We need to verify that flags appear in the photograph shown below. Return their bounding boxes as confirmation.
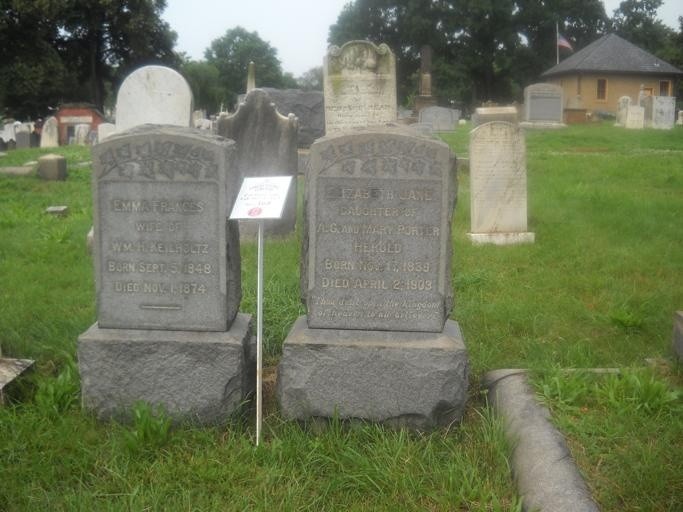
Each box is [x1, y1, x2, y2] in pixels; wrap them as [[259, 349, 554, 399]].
[[559, 34, 573, 52]]
